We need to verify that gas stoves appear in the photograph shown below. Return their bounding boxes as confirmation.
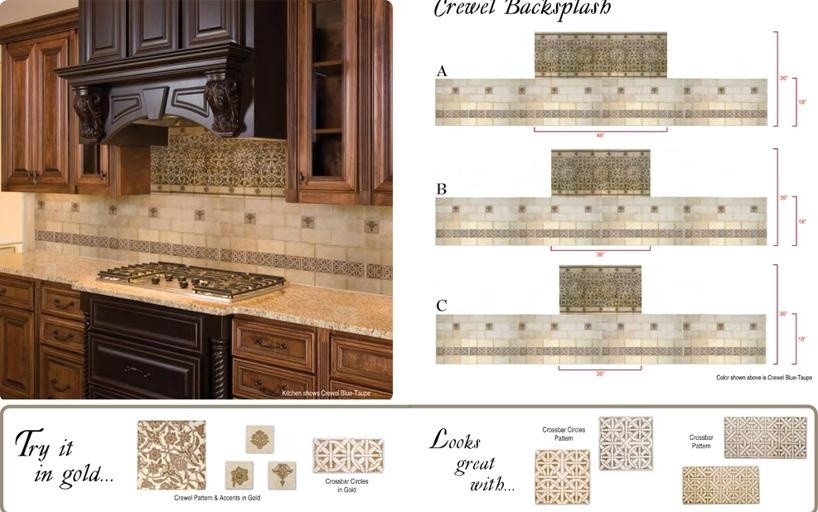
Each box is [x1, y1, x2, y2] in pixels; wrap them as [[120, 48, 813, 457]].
[[96, 260, 287, 304]]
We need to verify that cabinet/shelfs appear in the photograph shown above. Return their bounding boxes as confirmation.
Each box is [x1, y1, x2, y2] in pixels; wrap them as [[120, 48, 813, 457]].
[[0, 273, 392, 401], [0, 0, 393, 206]]
